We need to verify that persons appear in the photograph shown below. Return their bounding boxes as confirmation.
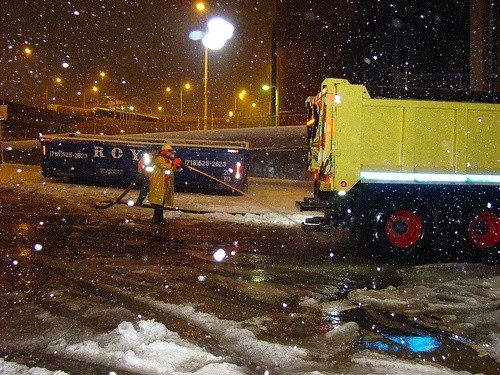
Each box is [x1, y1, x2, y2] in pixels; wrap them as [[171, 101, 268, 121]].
[[148, 143, 182, 227], [135, 148, 159, 208]]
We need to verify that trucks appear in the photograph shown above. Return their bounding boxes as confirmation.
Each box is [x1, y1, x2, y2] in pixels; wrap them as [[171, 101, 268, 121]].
[[295, 78, 500, 264]]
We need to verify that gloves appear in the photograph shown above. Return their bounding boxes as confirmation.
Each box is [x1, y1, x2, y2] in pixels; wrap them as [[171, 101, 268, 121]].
[[173, 158, 181, 167]]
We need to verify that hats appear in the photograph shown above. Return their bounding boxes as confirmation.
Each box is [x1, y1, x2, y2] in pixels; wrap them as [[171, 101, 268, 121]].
[[161, 144, 172, 150]]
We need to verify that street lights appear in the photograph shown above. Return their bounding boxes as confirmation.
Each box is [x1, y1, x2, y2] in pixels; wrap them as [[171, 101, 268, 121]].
[[196, 2, 210, 138]]
